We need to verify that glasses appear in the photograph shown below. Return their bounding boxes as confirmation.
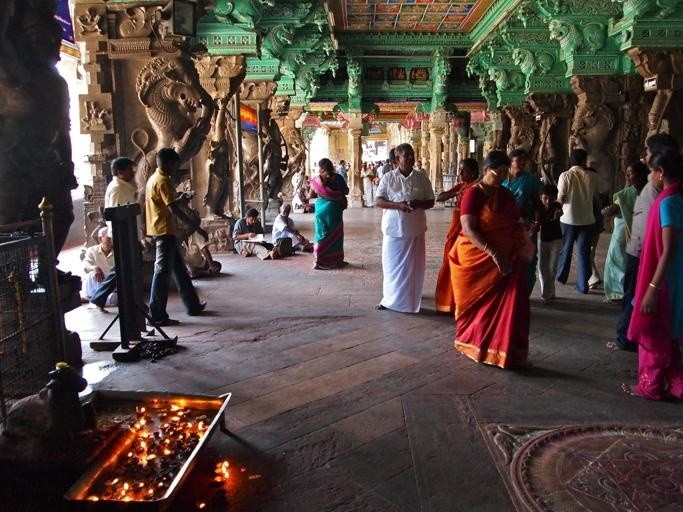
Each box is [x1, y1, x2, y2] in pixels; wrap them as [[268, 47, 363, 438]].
[[489, 168, 509, 179]]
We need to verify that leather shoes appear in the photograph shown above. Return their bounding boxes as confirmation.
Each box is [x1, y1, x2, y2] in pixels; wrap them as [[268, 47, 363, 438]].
[[147, 318, 180, 327], [187, 300, 207, 316]]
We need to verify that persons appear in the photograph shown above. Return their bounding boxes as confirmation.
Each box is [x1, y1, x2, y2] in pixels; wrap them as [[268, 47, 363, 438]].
[[529, 184, 564, 302], [374, 143, 435, 313], [413, 159, 428, 175], [308, 158, 349, 269], [291, 148, 399, 214], [555, 150, 610, 295], [435, 147, 543, 370], [271, 203, 314, 253], [82, 148, 222, 327], [601, 132, 683, 401], [232, 209, 279, 260]]
